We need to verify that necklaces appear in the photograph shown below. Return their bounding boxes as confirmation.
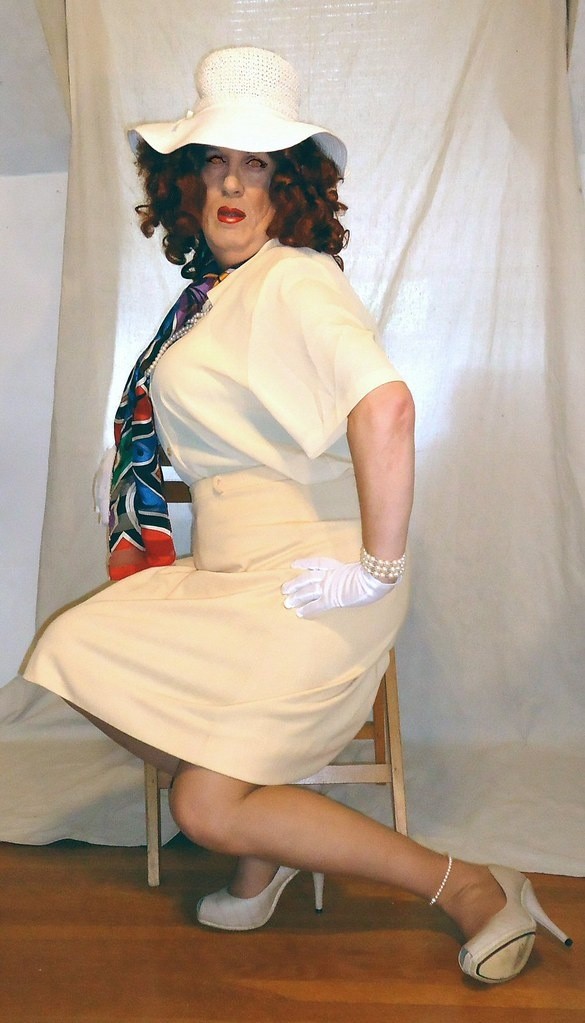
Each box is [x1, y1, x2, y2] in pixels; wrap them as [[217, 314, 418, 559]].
[[146, 304, 212, 377]]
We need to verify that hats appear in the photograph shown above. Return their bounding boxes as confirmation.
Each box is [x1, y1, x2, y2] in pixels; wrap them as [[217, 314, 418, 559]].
[[128, 46, 347, 183]]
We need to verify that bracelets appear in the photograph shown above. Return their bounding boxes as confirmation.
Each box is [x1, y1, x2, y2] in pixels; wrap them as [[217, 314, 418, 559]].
[[360, 546, 406, 578]]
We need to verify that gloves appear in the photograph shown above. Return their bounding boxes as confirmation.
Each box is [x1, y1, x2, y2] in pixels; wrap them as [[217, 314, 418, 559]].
[[280, 553, 402, 619]]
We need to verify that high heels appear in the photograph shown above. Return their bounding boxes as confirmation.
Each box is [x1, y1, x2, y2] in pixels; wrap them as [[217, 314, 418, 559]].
[[457, 868, 572, 983], [196, 865, 323, 930]]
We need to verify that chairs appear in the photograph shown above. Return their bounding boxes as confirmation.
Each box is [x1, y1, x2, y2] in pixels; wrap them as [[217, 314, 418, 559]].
[[147, 444, 406, 889]]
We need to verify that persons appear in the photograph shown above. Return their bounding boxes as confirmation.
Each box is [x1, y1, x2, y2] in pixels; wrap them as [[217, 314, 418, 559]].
[[22, 48, 571, 982]]
